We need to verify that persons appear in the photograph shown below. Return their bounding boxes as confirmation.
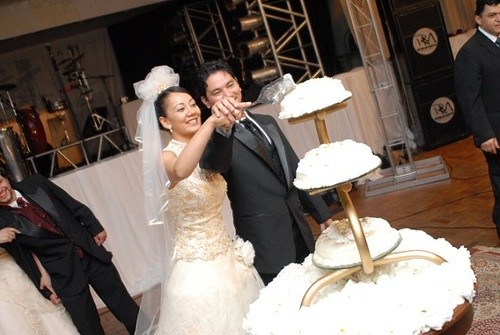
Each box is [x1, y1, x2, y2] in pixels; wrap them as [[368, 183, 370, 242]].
[[134, 65, 266, 335], [453, 0, 500, 241], [0, 227, 81, 334], [199, 58, 335, 287], [0, 167, 156, 335]]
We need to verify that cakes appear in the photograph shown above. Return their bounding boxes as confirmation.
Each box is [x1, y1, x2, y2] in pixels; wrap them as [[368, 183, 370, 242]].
[[313, 217, 400, 267], [278, 76, 352, 120], [293, 139, 382, 190]]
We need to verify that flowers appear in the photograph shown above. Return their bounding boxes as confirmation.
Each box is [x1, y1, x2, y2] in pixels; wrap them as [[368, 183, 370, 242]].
[[235, 238, 256, 265]]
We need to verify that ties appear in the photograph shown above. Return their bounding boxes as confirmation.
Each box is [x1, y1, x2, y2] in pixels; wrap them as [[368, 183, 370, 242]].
[[496, 38, 500, 45], [241, 117, 285, 175], [16, 198, 28, 206]]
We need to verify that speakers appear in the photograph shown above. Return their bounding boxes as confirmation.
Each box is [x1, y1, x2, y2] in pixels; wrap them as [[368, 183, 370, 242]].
[[376, 0, 473, 151]]
